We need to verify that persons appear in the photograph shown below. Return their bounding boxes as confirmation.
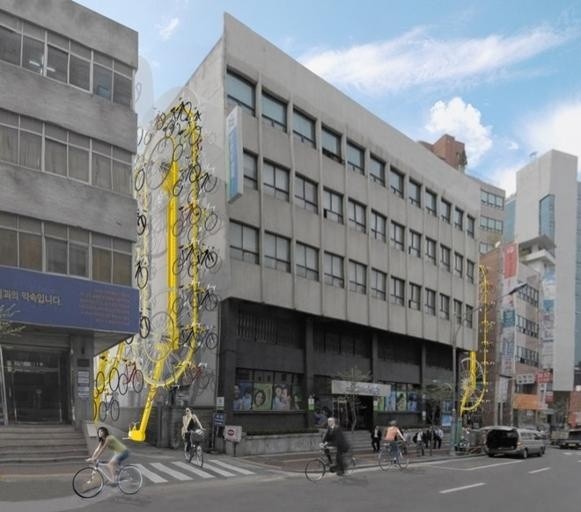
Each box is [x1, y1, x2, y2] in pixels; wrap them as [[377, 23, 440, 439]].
[[314, 410, 328, 441], [181, 407, 206, 454], [234, 384, 291, 410], [319, 417, 348, 475], [370, 420, 444, 467], [87, 428, 128, 485]]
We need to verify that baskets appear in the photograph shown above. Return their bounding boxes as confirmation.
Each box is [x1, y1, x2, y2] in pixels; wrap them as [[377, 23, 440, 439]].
[[190, 434, 206, 443]]
